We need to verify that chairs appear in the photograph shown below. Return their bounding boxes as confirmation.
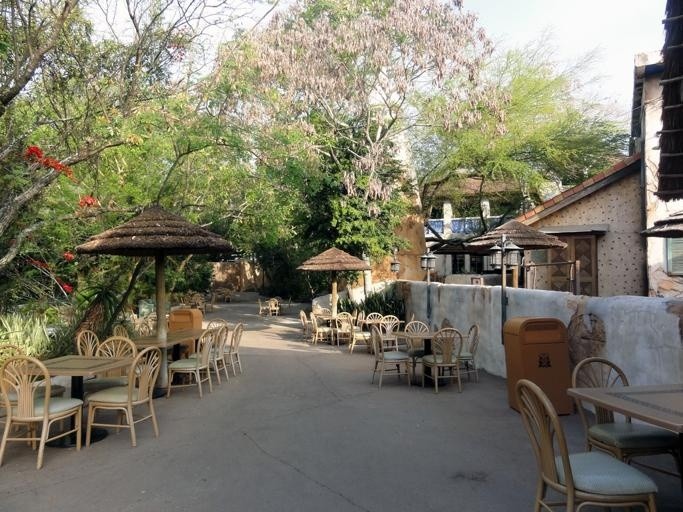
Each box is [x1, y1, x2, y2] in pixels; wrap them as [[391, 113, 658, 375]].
[[514, 378, 659, 512], [77, 318, 245, 448], [571, 357, 682, 491], [300, 308, 480, 394], [0, 344, 66, 447], [178, 285, 233, 315], [0, 354, 84, 470], [256, 295, 282, 318]]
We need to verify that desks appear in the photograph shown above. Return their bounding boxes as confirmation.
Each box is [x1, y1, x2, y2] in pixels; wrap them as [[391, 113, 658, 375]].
[[6, 354, 145, 447], [566, 384, 682, 434]]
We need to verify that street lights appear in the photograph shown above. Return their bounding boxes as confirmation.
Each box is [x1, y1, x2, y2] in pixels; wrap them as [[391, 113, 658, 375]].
[[389, 255, 401, 282], [417, 248, 437, 325], [488, 232, 523, 346]]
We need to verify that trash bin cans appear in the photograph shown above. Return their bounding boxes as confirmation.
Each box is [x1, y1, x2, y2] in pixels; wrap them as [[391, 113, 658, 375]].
[[503, 316, 575, 415], [168, 309, 202, 345]]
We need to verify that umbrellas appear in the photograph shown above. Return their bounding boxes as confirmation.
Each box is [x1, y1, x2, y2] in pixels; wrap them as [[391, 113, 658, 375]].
[[468, 218, 568, 289], [296, 247, 372, 340], [74, 204, 235, 388]]
